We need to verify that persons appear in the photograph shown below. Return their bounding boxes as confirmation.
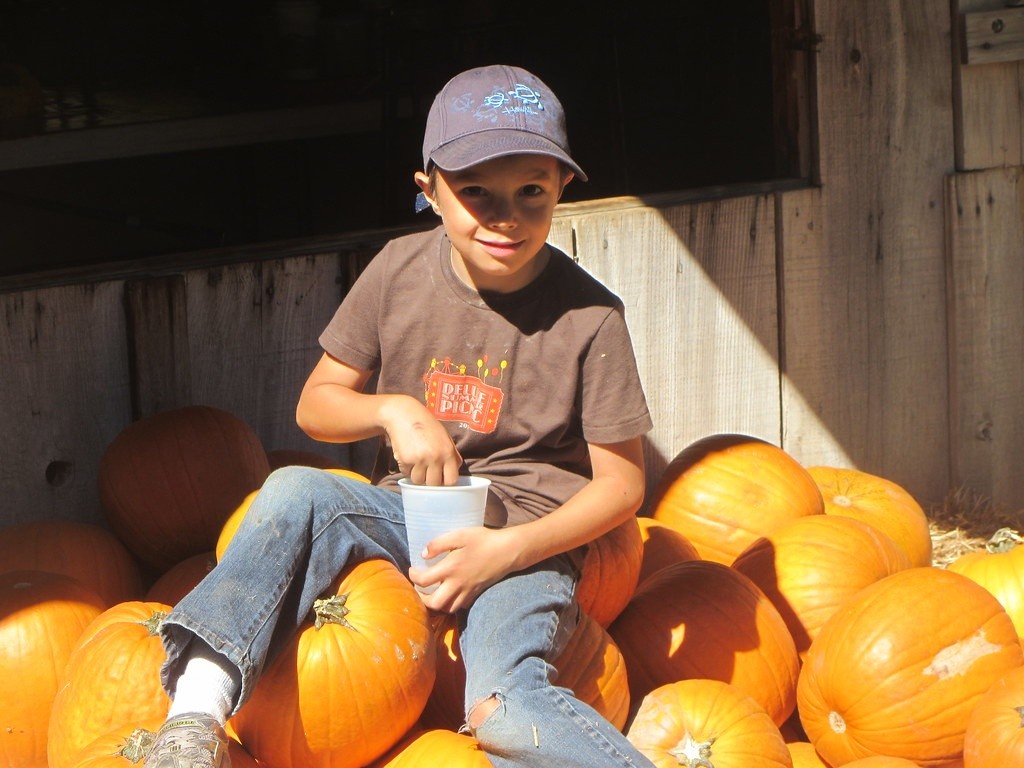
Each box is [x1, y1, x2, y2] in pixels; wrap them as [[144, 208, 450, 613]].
[[120, 65, 660, 768]]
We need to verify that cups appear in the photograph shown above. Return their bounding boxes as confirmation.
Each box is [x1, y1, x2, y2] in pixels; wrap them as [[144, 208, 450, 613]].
[[399, 476, 491, 593]]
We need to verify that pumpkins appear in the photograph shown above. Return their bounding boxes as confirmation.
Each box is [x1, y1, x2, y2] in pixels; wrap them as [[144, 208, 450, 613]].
[[0, 406, 1023, 767]]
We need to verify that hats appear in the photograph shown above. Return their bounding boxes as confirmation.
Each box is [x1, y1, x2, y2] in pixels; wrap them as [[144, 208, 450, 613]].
[[422, 64, 588, 182]]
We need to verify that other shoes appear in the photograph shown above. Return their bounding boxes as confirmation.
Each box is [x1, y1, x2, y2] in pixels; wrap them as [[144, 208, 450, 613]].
[[118, 712, 230, 768]]
